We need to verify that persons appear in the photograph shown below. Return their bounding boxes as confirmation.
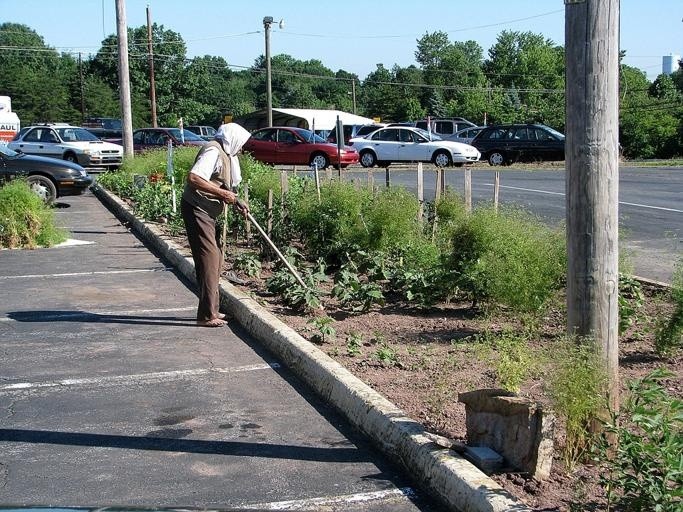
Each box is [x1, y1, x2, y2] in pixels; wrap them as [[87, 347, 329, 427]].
[[180, 123, 252, 327]]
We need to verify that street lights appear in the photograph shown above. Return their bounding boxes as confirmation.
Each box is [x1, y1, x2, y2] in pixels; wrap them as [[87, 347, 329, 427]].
[[262, 15, 286, 127]]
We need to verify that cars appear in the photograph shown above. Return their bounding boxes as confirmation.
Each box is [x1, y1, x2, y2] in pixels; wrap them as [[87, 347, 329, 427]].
[[0, 117, 218, 208]]
